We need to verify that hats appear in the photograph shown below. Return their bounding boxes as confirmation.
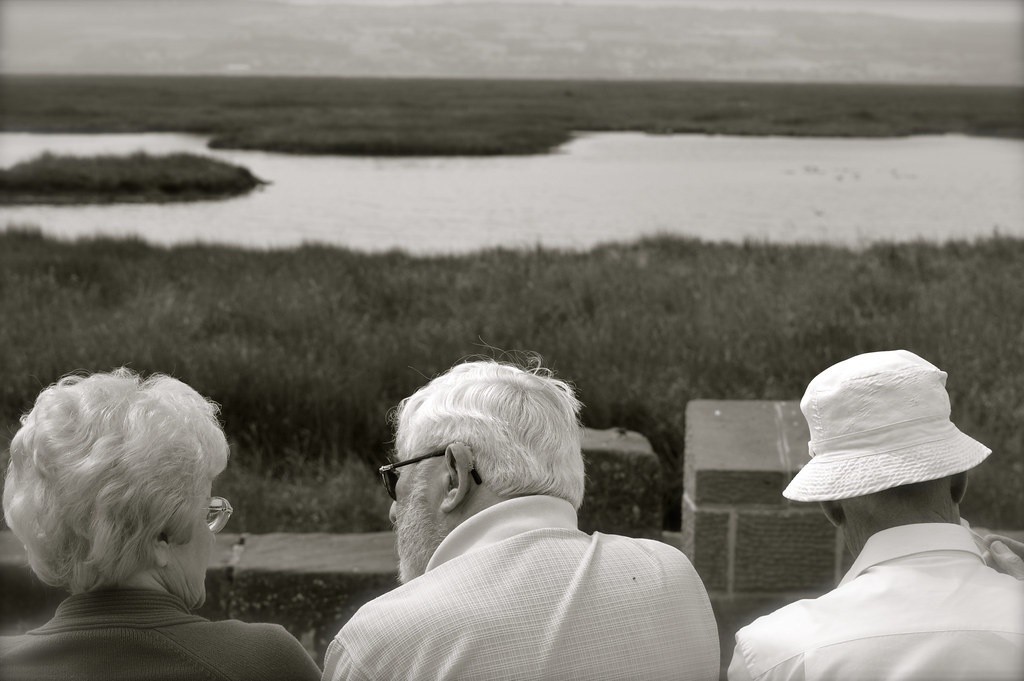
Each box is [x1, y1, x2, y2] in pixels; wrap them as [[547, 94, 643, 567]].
[[783, 349, 994, 501]]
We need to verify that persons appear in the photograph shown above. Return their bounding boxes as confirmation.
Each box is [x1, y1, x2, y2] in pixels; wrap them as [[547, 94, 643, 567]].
[[0, 366, 324, 681], [724, 345, 1022, 680], [311, 356, 721, 681]]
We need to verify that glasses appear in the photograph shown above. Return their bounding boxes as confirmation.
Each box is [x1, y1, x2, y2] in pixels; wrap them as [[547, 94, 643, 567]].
[[380, 448, 483, 501], [200, 496, 234, 533]]
